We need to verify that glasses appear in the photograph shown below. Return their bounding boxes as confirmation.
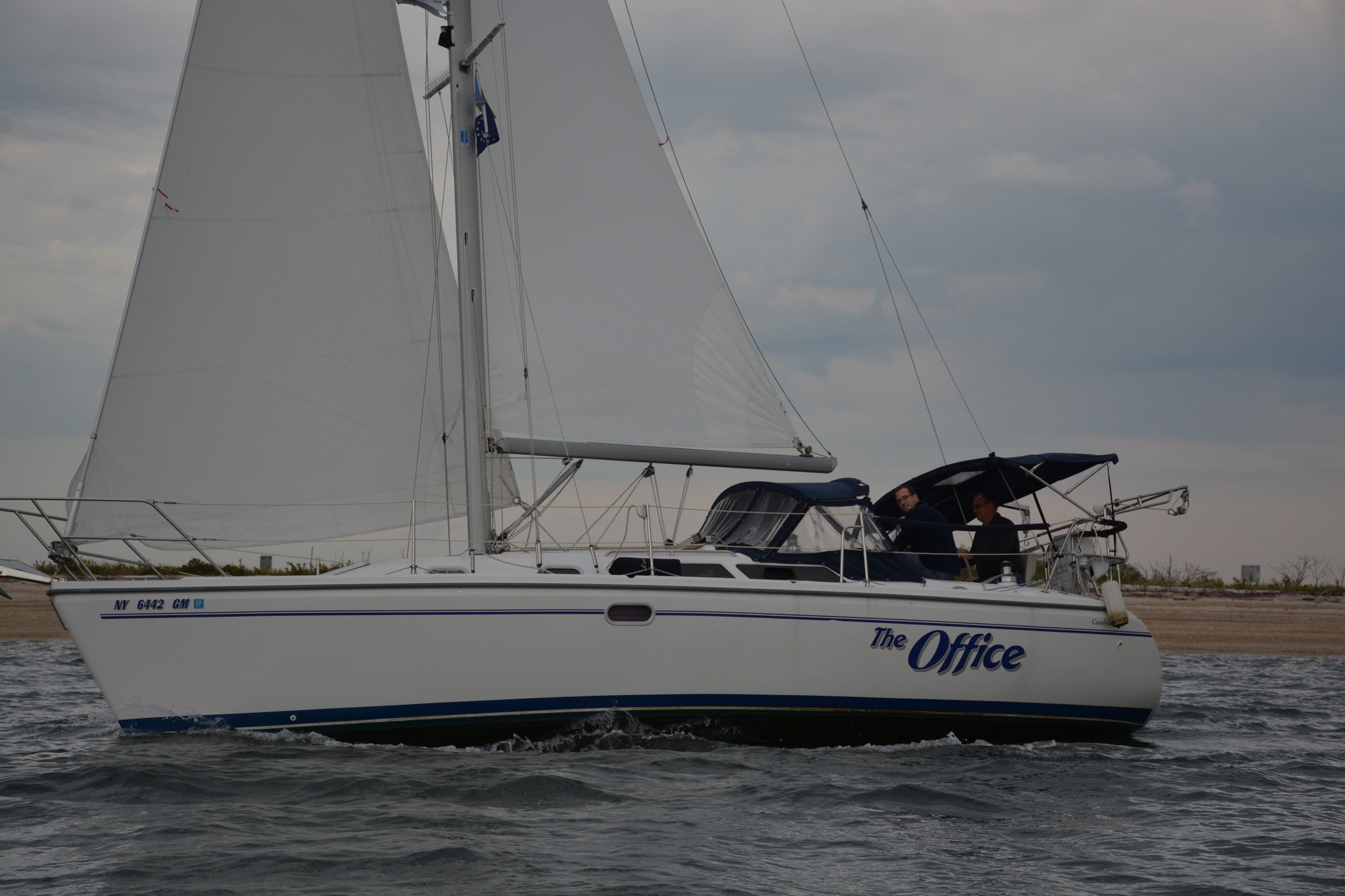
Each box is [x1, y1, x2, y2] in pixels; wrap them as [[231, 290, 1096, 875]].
[[896, 494, 913, 505], [973, 502, 992, 510]]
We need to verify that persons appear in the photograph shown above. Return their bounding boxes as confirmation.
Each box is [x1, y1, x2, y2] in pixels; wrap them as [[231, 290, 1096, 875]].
[[893, 485, 961, 580], [957, 492, 1024, 584]]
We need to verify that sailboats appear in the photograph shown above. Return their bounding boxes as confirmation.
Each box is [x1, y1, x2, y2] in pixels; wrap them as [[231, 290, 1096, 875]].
[[45, 1, 1193, 746]]
